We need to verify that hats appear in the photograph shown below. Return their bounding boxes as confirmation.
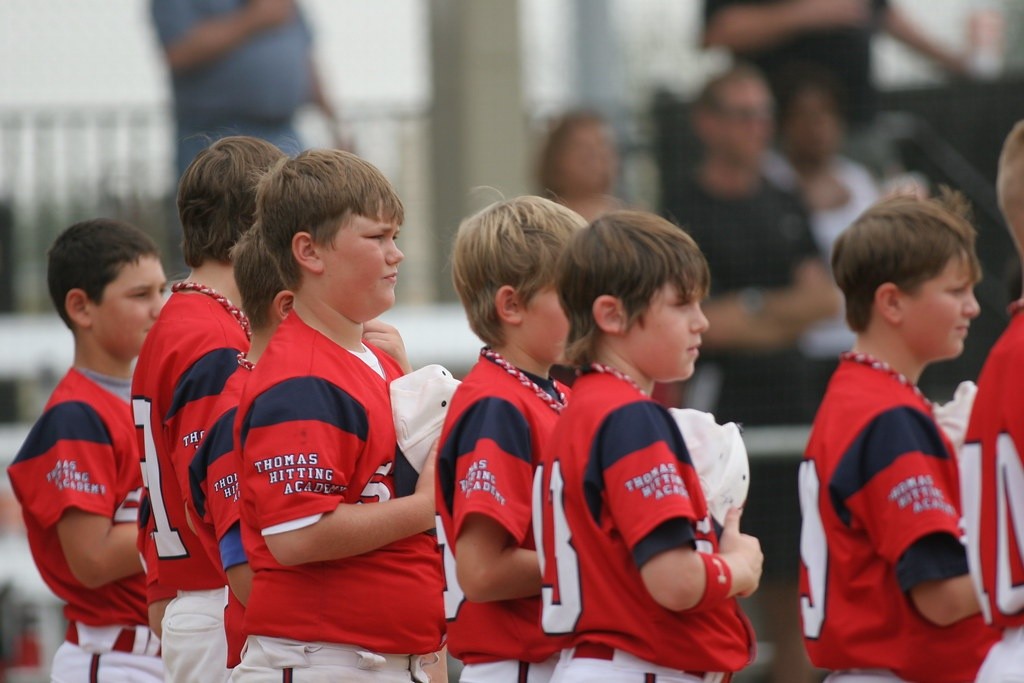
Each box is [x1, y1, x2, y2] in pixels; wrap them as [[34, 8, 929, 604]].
[[390, 363, 465, 536], [669, 405, 752, 543]]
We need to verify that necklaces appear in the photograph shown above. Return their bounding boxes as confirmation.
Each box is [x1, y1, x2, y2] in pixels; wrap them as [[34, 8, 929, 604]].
[[236, 352, 257, 370], [576, 362, 647, 397], [844, 352, 933, 405], [170, 282, 252, 341], [481, 346, 568, 410]]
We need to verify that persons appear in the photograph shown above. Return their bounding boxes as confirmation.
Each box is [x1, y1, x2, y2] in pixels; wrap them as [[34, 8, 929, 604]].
[[0, 0, 1024, 683], [801, 196, 981, 683]]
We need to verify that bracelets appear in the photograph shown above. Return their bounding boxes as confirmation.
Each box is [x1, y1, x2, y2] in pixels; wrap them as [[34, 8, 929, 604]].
[[683, 551, 730, 617]]
